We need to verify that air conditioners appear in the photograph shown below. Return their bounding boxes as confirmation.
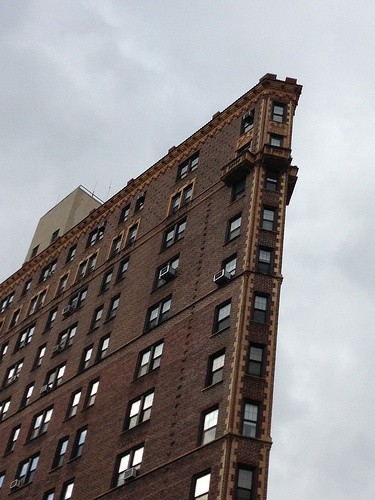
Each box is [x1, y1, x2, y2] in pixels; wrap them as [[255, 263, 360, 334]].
[[7, 375, 19, 385], [39, 384, 50, 395], [214, 269, 230, 283], [123, 468, 137, 480], [45, 272, 52, 279], [242, 112, 253, 120], [136, 192, 145, 200], [52, 342, 62, 354], [63, 305, 71, 315], [9, 478, 21, 491], [159, 265, 176, 281]]
